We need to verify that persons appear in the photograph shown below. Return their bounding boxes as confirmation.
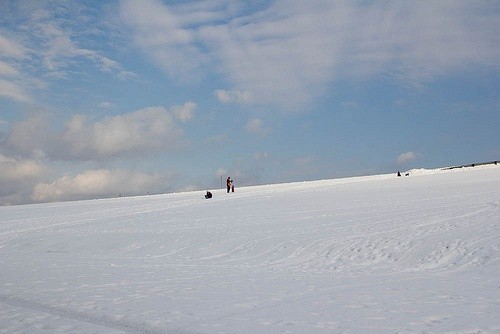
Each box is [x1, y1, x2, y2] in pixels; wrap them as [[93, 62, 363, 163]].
[[397, 160, 499, 177], [226, 176, 231, 193], [205, 191, 212, 199], [231, 179, 234, 192]]
